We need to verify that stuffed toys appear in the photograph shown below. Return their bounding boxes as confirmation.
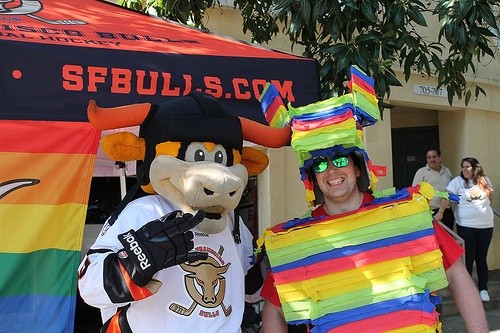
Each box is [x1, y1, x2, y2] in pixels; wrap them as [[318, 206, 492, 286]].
[[78, 99, 292, 333]]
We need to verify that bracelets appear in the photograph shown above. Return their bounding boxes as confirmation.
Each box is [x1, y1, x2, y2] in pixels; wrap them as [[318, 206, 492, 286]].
[[438, 209, 446, 215]]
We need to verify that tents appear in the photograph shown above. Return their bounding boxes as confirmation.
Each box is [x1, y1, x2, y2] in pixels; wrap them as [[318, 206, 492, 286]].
[[0, 0, 320, 333]]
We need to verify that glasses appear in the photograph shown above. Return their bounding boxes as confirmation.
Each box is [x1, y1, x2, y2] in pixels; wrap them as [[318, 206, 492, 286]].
[[314, 157, 348, 173]]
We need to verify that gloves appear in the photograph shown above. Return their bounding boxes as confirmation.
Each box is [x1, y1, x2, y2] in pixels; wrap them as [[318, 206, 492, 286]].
[[116, 209, 209, 287]]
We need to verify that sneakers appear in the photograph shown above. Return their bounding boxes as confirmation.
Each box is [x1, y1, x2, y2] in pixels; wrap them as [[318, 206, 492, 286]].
[[479, 290, 490, 301]]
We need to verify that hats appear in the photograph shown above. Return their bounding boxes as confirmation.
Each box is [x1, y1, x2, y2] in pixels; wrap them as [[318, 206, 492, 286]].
[[291, 93, 379, 201]]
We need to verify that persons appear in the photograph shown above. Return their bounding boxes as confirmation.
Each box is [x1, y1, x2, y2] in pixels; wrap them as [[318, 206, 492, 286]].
[[258, 64, 494, 333]]
